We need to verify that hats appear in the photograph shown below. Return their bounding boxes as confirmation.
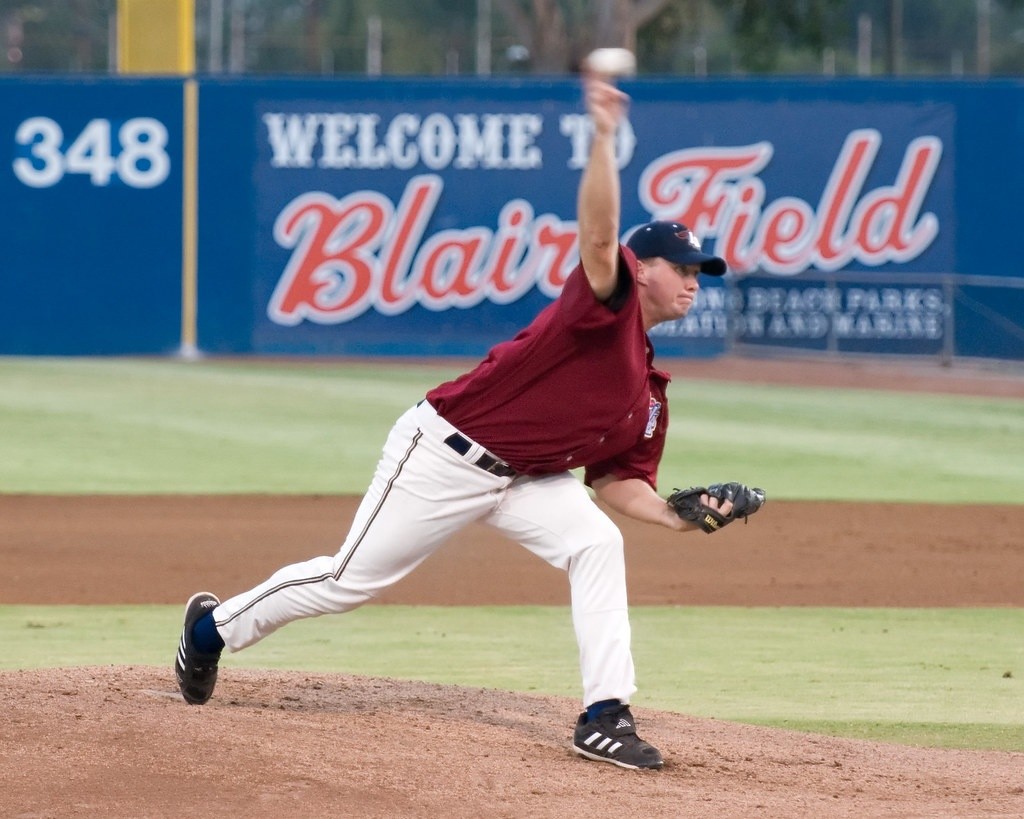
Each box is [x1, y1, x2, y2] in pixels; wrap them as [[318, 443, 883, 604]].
[[628, 220, 729, 277]]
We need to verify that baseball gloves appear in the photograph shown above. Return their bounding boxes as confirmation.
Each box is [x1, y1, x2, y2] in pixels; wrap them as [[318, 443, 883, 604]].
[[666, 482, 764, 534]]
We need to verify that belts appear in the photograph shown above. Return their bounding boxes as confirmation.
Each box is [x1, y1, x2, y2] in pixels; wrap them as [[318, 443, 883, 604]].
[[415, 399, 518, 478]]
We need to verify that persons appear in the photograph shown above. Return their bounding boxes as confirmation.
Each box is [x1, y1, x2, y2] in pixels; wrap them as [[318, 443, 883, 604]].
[[175, 59, 735, 769]]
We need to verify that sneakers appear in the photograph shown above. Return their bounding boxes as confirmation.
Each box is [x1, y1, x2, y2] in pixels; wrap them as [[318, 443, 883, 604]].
[[174, 592, 224, 705], [573, 702, 664, 773]]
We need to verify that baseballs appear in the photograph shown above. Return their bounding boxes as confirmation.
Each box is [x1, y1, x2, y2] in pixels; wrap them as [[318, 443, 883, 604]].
[[579, 43, 636, 79]]
